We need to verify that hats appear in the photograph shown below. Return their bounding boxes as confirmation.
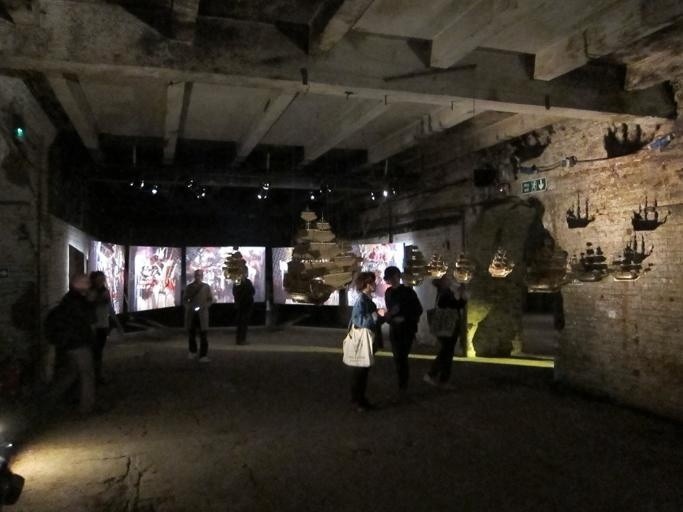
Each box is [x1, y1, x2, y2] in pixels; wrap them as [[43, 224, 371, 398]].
[[70, 274, 92, 290]]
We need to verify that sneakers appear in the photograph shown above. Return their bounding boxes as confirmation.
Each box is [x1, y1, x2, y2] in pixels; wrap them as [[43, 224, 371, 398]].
[[189, 352, 196, 359], [198, 355, 209, 362], [440, 384, 455, 390], [423, 374, 437, 386]]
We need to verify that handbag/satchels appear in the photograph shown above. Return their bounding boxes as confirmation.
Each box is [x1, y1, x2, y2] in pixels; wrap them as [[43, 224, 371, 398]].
[[342, 327, 376, 369], [426, 306, 458, 337]]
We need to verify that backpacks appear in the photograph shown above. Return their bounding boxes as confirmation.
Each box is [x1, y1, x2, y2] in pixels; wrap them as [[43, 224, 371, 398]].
[[44, 292, 80, 342]]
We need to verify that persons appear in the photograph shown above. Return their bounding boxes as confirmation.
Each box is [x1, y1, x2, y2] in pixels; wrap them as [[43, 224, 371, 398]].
[[423, 274, 469, 389], [384, 267, 423, 404], [91, 269, 125, 383], [343, 271, 400, 413], [232, 279, 256, 345], [182, 270, 212, 363], [39, 273, 104, 418]]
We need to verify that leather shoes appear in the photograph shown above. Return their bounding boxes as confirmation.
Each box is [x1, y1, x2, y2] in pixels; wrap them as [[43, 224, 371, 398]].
[[349, 401, 364, 413]]
